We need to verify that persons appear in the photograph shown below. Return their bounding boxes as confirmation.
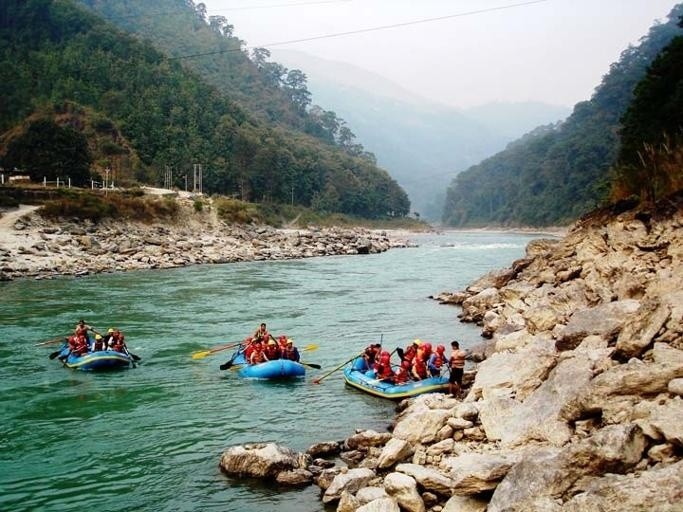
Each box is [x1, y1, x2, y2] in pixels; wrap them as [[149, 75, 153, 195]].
[[61, 318, 124, 353], [242, 323, 303, 364], [362, 341, 465, 401]]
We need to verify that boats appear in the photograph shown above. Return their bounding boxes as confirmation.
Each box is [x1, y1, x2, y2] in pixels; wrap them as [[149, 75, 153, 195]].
[[232, 346, 306, 380], [342, 355, 460, 399], [57, 334, 131, 371]]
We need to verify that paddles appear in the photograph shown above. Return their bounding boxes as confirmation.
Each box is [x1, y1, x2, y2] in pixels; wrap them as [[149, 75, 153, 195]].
[[120, 351, 140, 361], [299, 363, 322, 369], [57, 351, 74, 361], [298, 343, 319, 353], [123, 346, 137, 369], [220, 363, 249, 369], [397, 347, 407, 368], [49, 346, 68, 360], [193, 341, 245, 358], [35, 338, 65, 346]]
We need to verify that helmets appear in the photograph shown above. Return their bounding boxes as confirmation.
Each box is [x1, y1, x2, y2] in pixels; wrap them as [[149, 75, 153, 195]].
[[69, 336, 85, 343], [95, 329, 124, 340], [416, 359, 424, 366], [247, 337, 262, 350], [413, 338, 444, 354], [402, 360, 410, 369], [381, 351, 389, 363], [280, 336, 292, 346]]
